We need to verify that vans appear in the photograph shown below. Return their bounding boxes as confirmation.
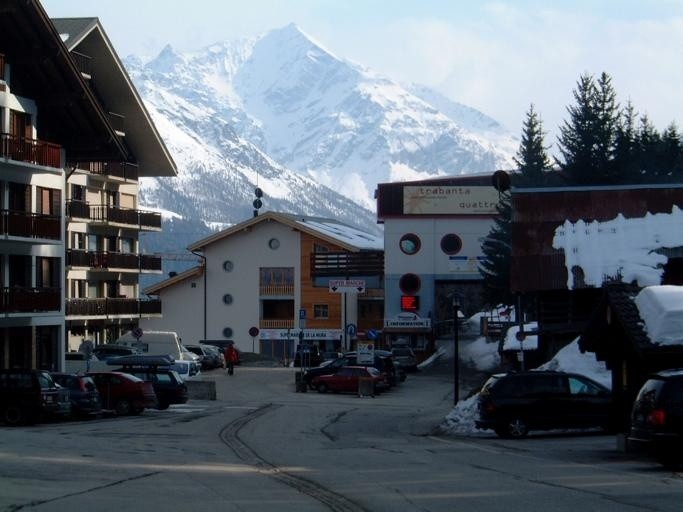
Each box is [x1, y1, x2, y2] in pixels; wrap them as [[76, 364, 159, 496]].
[[626, 368, 683, 467]]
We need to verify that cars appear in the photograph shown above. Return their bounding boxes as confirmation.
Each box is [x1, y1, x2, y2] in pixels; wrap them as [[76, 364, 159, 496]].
[[0, 329, 226, 426], [294, 345, 418, 394], [470, 371, 616, 439]]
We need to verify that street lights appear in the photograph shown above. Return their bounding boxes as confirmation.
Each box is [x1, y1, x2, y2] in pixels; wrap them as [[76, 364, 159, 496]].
[[446, 289, 467, 407]]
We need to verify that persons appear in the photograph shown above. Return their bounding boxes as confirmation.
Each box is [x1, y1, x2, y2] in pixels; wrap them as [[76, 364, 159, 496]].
[[223, 339, 238, 376]]
